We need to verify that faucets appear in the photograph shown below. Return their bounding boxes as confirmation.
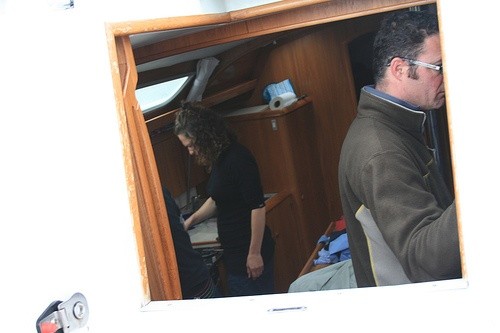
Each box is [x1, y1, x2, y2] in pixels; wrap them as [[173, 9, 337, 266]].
[[192, 194, 201, 210]]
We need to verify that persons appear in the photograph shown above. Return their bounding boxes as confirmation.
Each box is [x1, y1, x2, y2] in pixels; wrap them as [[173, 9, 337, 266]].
[[337, 7, 462, 289], [174, 102, 275, 300], [158, 181, 219, 299]]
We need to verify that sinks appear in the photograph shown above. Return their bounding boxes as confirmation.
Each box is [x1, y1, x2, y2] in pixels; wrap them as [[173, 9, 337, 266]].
[[207, 193, 273, 222]]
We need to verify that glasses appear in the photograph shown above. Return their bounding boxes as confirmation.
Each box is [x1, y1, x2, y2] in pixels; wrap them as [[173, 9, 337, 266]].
[[386, 56, 442, 77]]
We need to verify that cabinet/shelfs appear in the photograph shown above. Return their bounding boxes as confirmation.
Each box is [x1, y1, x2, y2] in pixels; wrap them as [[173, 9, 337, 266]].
[[187, 97, 354, 299]]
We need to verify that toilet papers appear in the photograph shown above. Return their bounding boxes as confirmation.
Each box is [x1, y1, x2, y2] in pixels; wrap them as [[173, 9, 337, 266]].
[[269, 92, 297, 111]]
